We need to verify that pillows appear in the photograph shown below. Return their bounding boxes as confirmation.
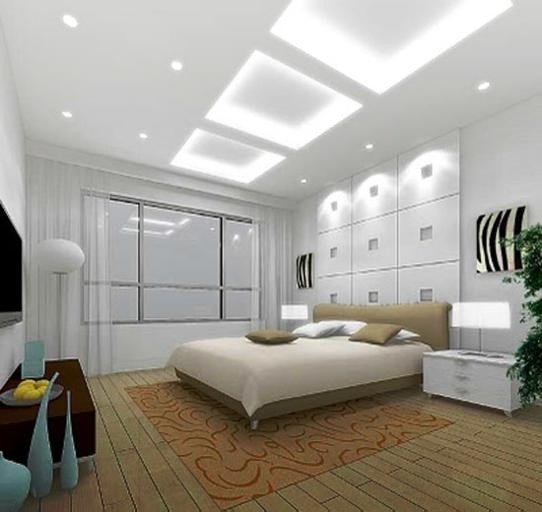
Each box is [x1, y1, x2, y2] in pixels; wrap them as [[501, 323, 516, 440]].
[[292, 320, 421, 346], [245, 329, 300, 345]]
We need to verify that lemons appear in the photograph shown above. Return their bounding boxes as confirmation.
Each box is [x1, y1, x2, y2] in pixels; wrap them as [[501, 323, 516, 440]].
[[14, 378, 49, 401]]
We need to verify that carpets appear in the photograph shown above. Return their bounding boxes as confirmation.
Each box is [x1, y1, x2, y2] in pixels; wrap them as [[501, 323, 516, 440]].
[[124, 379, 457, 512]]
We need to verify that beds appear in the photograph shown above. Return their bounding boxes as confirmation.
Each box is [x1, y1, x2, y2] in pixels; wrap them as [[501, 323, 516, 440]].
[[165, 302, 453, 432]]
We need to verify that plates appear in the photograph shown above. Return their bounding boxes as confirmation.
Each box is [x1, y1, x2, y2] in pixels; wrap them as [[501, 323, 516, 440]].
[[1, 385, 64, 406]]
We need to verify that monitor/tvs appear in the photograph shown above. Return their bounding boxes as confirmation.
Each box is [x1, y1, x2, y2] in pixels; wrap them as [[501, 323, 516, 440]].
[[0, 201, 23, 328]]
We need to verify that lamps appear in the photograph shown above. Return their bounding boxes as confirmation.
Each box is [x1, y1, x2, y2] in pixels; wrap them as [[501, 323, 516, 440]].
[[451, 301, 511, 358], [34, 238, 85, 360], [281, 304, 308, 333]]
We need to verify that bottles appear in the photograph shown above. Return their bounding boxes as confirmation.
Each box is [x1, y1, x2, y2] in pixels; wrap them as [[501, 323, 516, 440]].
[[60, 391, 81, 489], [0, 451, 31, 512], [24, 371, 60, 498]]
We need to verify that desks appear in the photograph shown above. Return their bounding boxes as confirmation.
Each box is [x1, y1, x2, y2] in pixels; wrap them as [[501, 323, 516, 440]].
[[0, 357, 98, 478]]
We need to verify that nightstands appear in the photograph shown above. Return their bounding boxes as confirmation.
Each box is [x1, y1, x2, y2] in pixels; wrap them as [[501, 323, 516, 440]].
[[421, 348, 537, 419]]
